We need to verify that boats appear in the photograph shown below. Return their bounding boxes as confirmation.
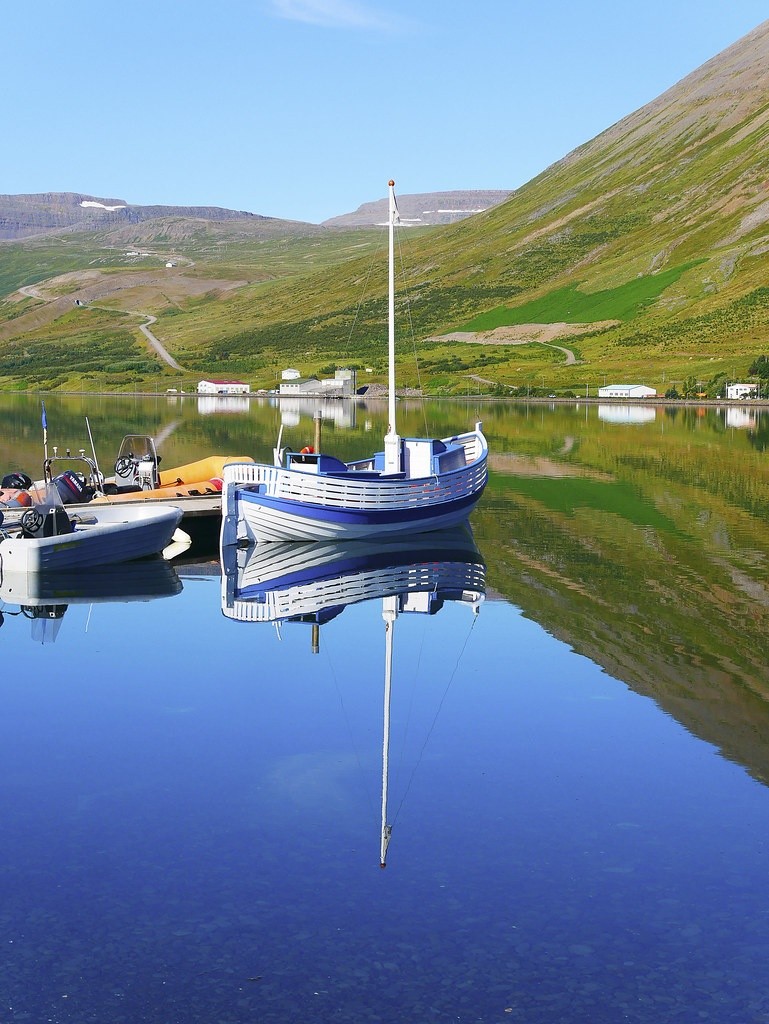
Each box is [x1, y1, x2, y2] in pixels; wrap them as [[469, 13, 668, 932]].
[[11, 556, 184, 604], [10, 505, 181, 568], [0, 453, 254, 504]]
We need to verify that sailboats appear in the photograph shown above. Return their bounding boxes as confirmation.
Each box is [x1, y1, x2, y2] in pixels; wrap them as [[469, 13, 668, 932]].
[[200, 521, 488, 867], [220, 181, 491, 544]]
